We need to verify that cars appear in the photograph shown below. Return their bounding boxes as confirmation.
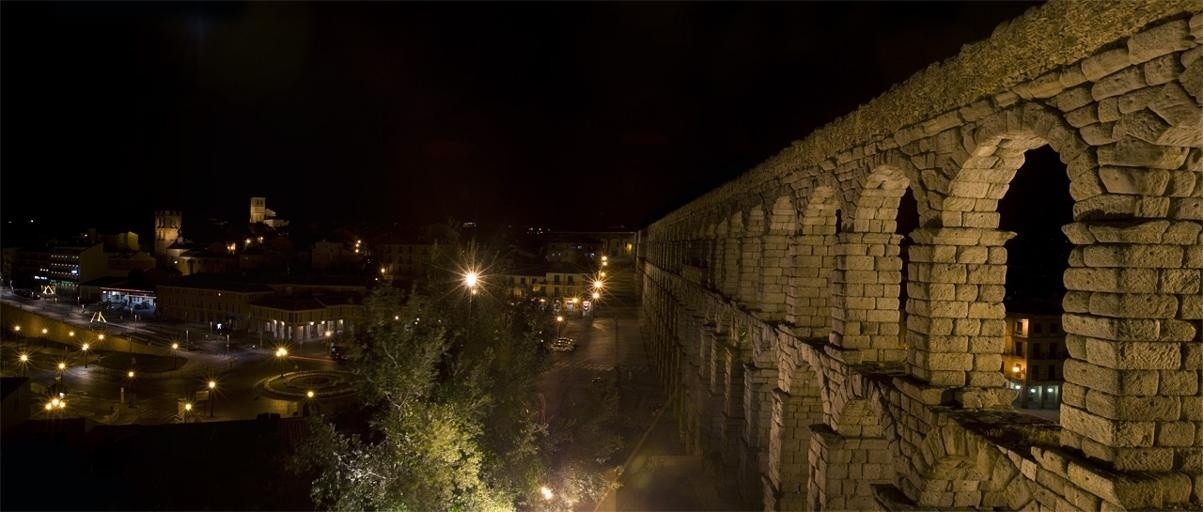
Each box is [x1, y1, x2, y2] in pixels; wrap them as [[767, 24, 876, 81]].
[[550, 336, 576, 353]]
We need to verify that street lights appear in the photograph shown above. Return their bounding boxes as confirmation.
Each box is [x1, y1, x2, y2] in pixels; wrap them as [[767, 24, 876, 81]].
[[208, 381, 216, 417], [58, 362, 66, 392], [557, 316, 563, 336], [274, 346, 288, 380], [14, 325, 106, 354], [19, 354, 28, 375], [128, 371, 136, 409], [81, 342, 89, 369], [45, 398, 65, 418], [171, 342, 178, 370], [593, 292, 599, 318]]
